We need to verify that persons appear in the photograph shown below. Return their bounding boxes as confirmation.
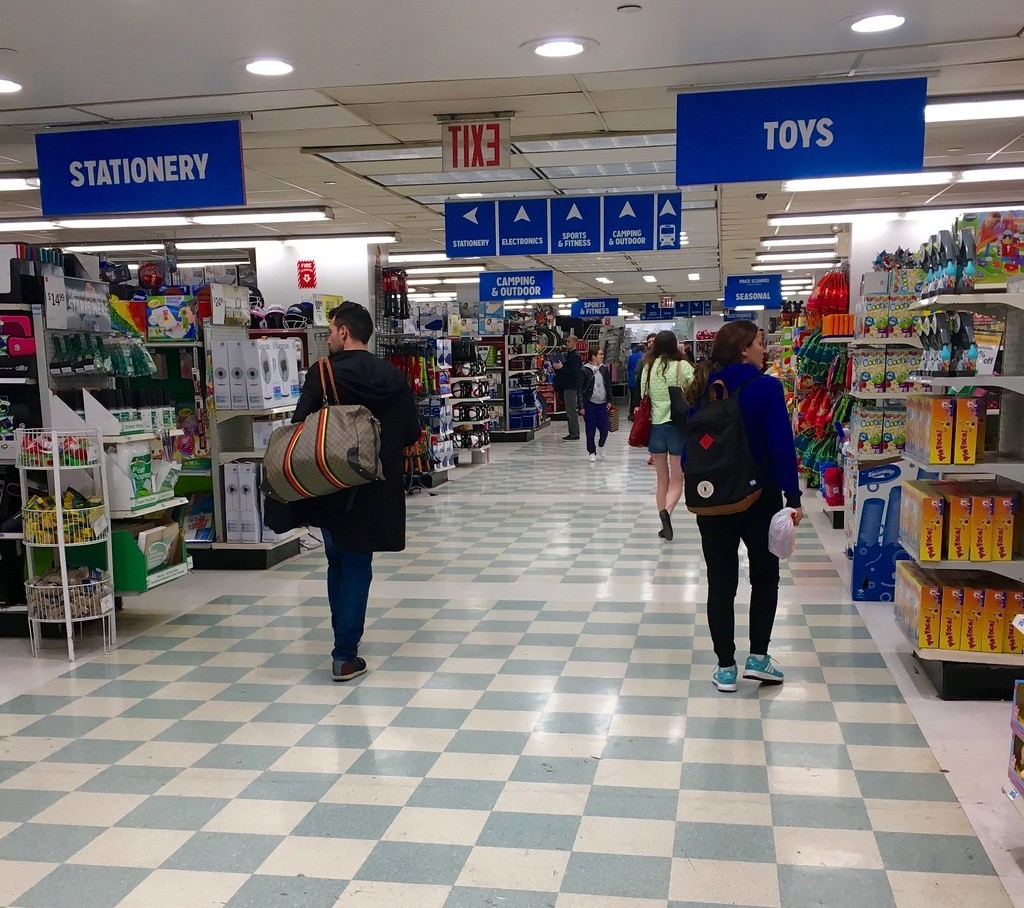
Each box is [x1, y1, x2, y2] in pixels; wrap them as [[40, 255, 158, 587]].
[[681, 320, 803, 692], [640, 331, 695, 541], [685, 345, 695, 363], [759, 329, 768, 372], [292, 301, 421, 681], [553, 335, 582, 442], [627, 344, 647, 421], [577, 347, 613, 462], [636, 333, 656, 464]]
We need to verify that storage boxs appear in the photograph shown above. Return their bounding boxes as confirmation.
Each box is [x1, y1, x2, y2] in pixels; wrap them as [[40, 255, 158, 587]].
[[251, 418, 292, 454], [906, 396, 986, 465], [224, 460, 294, 542], [895, 477, 1024, 653], [211, 338, 300, 411]]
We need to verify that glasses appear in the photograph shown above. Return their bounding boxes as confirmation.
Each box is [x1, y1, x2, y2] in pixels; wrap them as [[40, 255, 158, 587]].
[[647, 340, 654, 344], [597, 352, 604, 355]]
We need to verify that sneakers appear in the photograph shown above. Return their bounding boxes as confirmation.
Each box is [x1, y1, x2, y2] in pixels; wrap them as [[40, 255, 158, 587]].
[[742, 652, 784, 682], [712, 664, 738, 692]]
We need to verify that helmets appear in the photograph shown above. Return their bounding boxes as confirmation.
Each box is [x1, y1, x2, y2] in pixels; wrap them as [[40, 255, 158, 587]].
[[99, 257, 313, 328]]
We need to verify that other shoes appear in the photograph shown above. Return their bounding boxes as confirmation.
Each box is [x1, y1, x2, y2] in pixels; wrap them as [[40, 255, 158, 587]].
[[646, 457, 655, 465], [660, 509, 673, 541], [597, 438, 605, 458], [562, 435, 580, 441], [589, 453, 597, 462], [332, 657, 367, 681], [659, 530, 666, 539]]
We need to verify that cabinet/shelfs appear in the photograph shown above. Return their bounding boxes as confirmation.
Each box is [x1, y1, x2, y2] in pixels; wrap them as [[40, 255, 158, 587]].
[[0, 302, 194, 634], [905, 277, 1024, 666], [444, 333, 551, 451], [843, 293, 925, 558], [119, 320, 306, 568]]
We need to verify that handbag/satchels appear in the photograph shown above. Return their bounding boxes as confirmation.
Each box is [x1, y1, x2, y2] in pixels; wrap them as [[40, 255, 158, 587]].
[[576, 391, 587, 417], [608, 406, 619, 433], [264, 355, 386, 503], [768, 507, 799, 560], [628, 365, 651, 448], [668, 359, 688, 424]]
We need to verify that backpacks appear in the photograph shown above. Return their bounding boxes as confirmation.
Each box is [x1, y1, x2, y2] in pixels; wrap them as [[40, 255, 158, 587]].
[[680, 373, 777, 515]]
